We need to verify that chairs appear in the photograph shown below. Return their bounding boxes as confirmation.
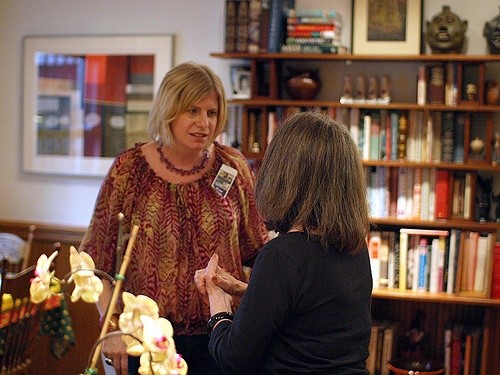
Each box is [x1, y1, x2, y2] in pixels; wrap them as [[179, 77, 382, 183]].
[[0, 241, 62, 375]]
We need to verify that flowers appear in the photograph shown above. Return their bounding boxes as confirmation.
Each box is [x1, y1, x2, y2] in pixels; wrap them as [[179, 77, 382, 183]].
[[397, 311, 434, 375], [29, 245, 188, 375]]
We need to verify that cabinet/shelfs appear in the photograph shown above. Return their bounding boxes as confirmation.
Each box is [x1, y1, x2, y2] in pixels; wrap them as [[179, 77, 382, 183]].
[[210, 53, 500, 306]]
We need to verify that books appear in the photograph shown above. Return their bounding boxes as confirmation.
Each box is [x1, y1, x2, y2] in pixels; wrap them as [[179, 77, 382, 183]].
[[225, 0, 500, 375]]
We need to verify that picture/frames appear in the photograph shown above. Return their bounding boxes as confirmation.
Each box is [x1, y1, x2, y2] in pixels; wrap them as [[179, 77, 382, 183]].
[[18, 32, 177, 180], [350, 0, 424, 55]]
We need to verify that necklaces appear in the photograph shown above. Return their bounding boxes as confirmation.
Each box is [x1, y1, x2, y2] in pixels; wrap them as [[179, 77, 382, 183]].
[[156, 139, 209, 175]]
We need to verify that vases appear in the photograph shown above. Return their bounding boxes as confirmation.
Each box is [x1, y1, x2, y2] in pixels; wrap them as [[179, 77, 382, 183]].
[[386, 361, 446, 375]]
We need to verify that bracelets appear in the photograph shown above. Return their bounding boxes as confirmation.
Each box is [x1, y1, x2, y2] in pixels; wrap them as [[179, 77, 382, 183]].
[[99, 313, 119, 333], [207, 311, 234, 336]]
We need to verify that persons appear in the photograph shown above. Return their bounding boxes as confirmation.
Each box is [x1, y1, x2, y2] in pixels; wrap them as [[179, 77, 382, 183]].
[[194, 113, 372, 375], [81, 63, 270, 375]]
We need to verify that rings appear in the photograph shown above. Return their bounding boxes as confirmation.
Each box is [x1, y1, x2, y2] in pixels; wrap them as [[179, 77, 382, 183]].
[[104, 358, 113, 366]]
